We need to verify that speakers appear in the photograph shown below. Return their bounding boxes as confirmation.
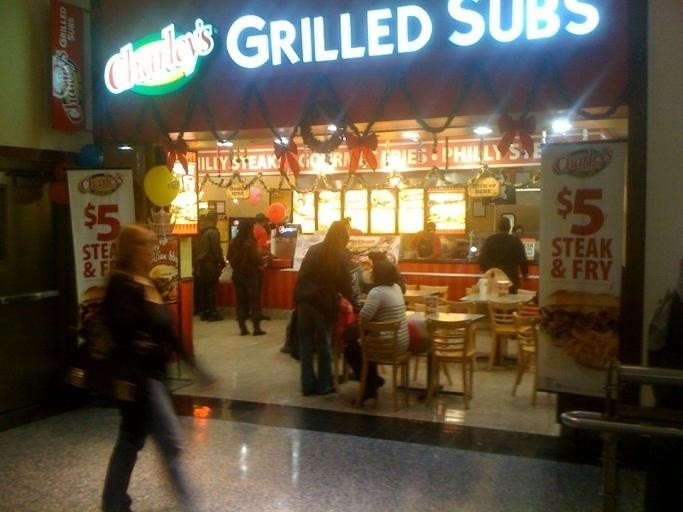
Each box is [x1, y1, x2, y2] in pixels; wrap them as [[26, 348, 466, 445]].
[[482, 184, 515, 205]]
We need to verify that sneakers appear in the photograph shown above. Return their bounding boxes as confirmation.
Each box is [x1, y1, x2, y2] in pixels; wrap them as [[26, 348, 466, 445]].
[[206, 311, 224, 323]]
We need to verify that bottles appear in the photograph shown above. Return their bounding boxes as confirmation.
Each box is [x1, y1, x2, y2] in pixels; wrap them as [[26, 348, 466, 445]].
[[471, 285, 477, 296]]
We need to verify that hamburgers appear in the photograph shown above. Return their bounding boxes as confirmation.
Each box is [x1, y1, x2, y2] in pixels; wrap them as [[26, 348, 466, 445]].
[[538, 290, 621, 347], [80, 286, 107, 312]]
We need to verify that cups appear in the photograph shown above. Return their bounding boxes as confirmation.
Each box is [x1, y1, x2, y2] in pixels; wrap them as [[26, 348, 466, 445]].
[[478, 278, 489, 299], [413, 303, 426, 319], [495, 279, 510, 297]]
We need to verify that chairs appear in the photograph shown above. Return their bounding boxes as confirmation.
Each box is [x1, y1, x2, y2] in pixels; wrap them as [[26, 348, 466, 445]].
[[322, 282, 549, 415]]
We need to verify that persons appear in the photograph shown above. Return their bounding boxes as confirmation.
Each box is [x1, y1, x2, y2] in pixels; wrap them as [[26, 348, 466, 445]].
[[194, 209, 225, 322], [279, 215, 410, 407], [94, 222, 218, 512], [225, 218, 266, 336], [409, 220, 442, 260], [191, 214, 206, 316], [510, 224, 523, 237], [251, 213, 271, 251], [478, 216, 529, 340]]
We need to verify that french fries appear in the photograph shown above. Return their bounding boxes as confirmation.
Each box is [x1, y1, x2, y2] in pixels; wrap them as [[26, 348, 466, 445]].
[[565, 330, 620, 368]]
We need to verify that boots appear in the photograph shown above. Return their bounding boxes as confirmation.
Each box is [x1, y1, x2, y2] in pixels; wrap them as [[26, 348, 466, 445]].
[[252, 321, 267, 336], [237, 321, 250, 335]]
